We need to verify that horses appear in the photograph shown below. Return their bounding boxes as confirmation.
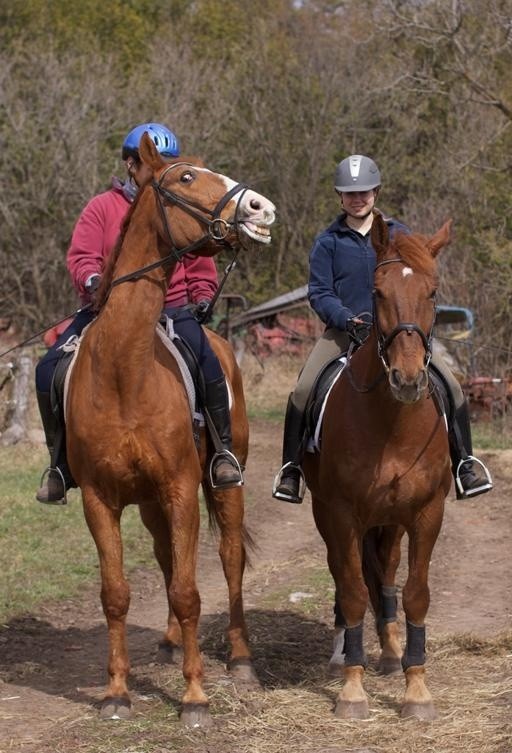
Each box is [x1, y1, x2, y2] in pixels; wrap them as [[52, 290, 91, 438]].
[[58, 130, 264, 730], [295, 208, 454, 722]]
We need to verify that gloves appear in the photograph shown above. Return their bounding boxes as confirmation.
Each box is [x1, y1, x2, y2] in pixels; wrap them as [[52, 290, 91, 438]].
[[195, 300, 215, 324], [349, 319, 369, 338]]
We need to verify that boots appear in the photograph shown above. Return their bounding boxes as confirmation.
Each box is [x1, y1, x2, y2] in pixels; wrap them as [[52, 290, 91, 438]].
[[447, 388, 491, 501], [273, 388, 308, 502], [201, 370, 242, 485], [32, 390, 80, 501]]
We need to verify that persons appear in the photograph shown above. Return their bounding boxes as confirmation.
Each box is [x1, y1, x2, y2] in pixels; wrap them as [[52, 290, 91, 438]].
[[272, 154, 493, 504], [35, 124, 243, 503]]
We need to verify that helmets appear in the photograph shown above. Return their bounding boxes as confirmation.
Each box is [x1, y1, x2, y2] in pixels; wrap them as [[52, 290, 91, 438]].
[[118, 121, 182, 161], [333, 154, 382, 195]]
